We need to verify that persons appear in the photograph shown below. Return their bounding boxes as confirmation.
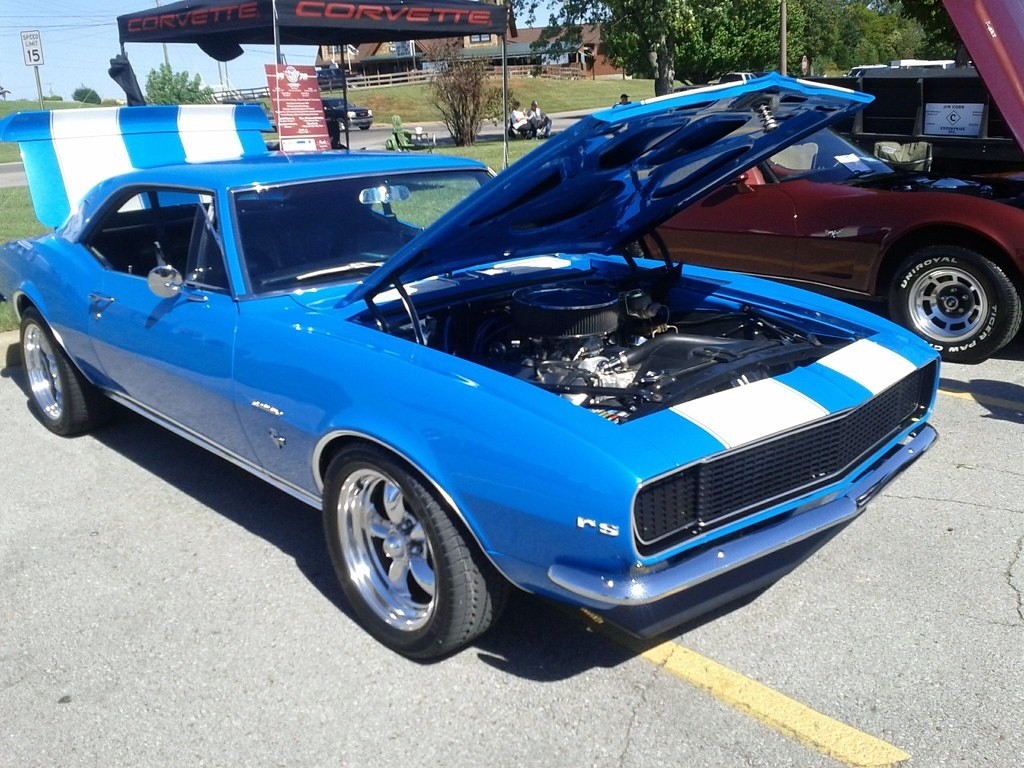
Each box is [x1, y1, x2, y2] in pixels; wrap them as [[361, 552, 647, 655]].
[[612, 94, 631, 108], [510, 100, 552, 140]]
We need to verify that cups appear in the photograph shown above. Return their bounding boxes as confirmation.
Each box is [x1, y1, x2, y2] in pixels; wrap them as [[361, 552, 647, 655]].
[[415, 127, 422, 133]]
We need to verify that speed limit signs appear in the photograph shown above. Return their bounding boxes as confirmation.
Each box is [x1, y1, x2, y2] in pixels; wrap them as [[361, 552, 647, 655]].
[[20, 30, 44, 66]]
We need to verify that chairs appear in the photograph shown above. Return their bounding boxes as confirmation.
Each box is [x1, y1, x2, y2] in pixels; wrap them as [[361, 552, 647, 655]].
[[391, 114, 437, 154]]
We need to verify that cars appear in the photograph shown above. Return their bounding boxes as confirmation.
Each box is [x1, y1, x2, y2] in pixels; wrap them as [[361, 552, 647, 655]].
[[0, 70, 940, 665], [842, 64, 887, 76], [319, 97, 374, 131], [626, 0, 1024, 366], [221, 99, 277, 132], [315, 71, 347, 92], [719, 72, 758, 81]]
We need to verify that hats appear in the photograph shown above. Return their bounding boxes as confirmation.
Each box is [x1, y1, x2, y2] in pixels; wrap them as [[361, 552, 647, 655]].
[[531, 100, 538, 106], [621, 93, 630, 98]]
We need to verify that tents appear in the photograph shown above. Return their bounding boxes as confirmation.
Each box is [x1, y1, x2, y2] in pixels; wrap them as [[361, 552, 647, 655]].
[[116, 0, 508, 169]]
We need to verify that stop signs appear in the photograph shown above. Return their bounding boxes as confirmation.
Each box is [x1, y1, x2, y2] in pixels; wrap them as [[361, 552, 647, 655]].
[[802, 55, 808, 75]]
